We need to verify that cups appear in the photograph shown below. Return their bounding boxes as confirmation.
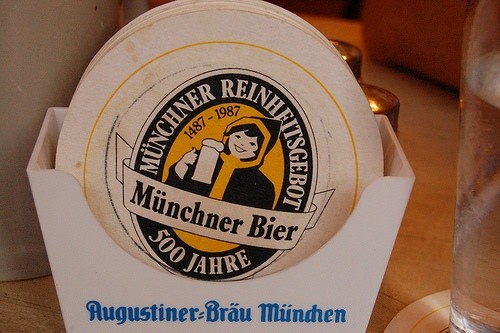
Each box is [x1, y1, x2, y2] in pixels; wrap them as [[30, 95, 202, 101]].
[[448, 0, 500, 333]]
